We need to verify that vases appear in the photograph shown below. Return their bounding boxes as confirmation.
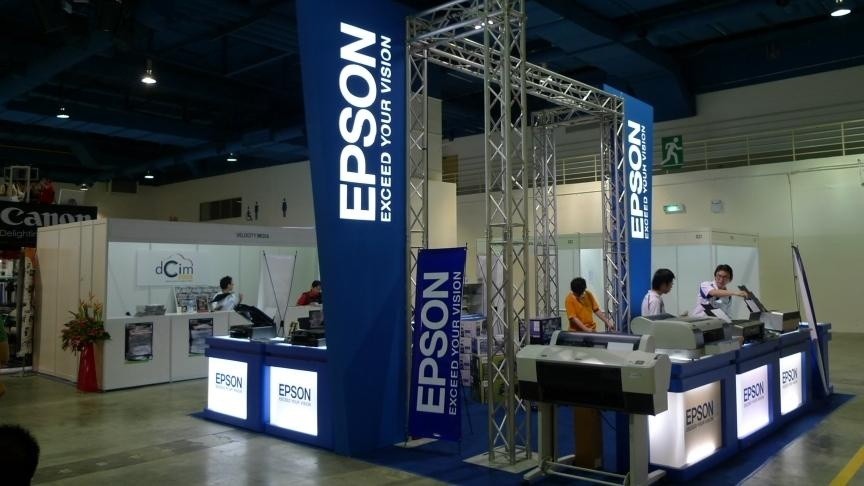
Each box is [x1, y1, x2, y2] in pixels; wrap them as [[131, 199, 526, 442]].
[[76, 344, 99, 393]]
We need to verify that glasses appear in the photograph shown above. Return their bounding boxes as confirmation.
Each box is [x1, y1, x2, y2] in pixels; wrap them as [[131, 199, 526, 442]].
[[716, 274, 728, 280]]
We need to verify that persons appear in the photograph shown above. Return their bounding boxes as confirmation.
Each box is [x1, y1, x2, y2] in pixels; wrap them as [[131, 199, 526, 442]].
[[296, 280, 322, 308], [659, 137, 683, 165], [694, 264, 748, 318], [641, 268, 676, 316], [211, 276, 244, 311], [565, 277, 614, 333], [0, 174, 55, 203], [0, 424, 44, 486]]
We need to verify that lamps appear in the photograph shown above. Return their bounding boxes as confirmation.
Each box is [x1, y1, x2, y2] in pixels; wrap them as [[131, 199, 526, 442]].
[[226, 152, 238, 163], [143, 168, 155, 179], [830, 0, 852, 18], [56, 103, 70, 118], [140, 58, 157, 85]]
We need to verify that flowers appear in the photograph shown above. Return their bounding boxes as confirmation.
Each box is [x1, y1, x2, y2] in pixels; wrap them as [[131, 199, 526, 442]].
[[60, 292, 112, 355]]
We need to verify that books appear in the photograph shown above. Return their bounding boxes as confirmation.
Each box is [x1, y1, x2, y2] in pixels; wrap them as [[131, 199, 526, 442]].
[[134, 300, 166, 316]]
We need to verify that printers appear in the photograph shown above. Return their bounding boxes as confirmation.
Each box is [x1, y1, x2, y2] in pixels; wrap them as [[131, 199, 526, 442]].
[[291, 309, 327, 347], [631, 313, 740, 355], [701, 303, 764, 345], [516, 330, 671, 416], [739, 284, 800, 335], [229, 303, 276, 340]]
